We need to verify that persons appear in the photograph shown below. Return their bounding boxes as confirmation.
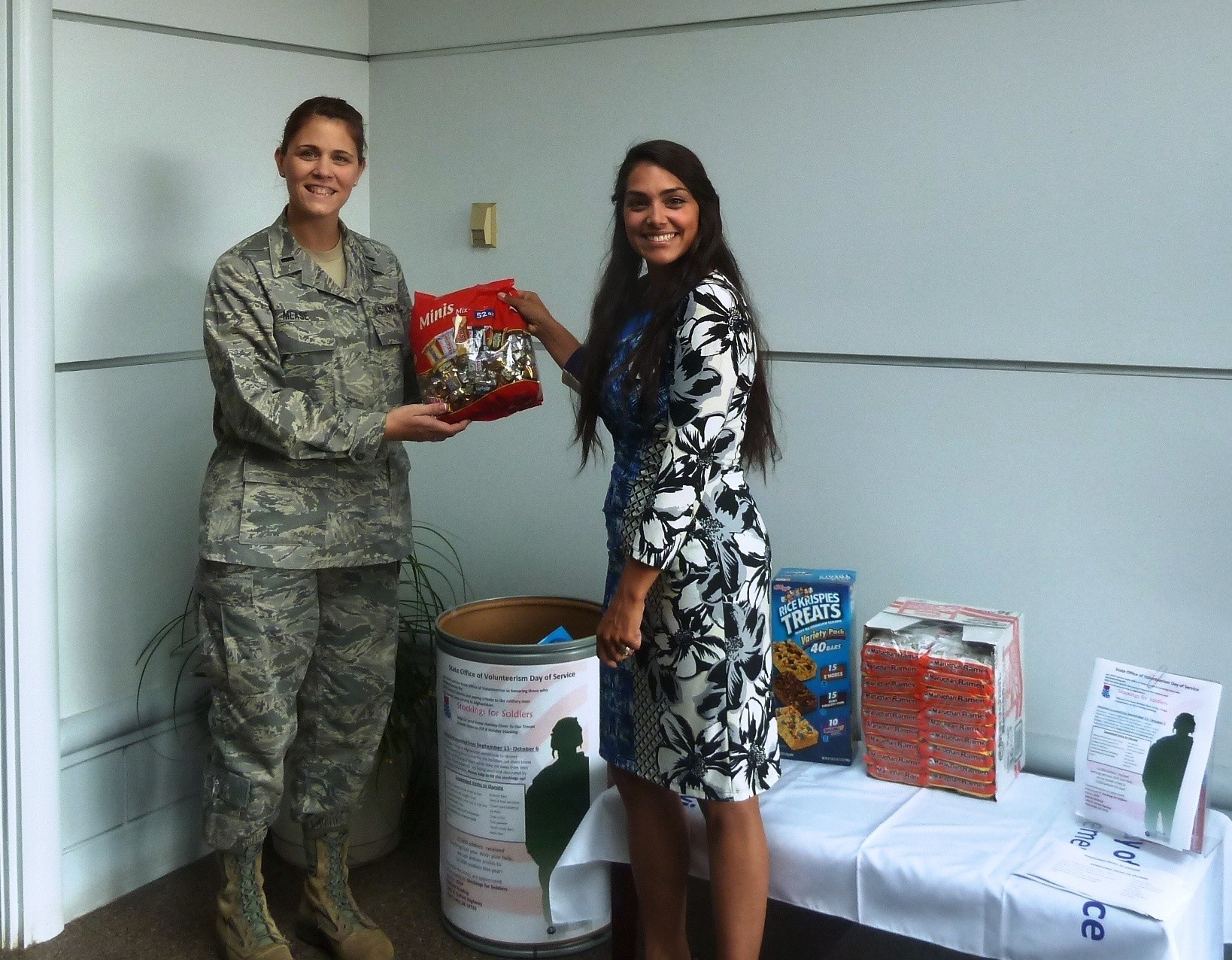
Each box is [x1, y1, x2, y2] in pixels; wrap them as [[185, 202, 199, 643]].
[[501, 141, 783, 960], [197, 95, 471, 960]]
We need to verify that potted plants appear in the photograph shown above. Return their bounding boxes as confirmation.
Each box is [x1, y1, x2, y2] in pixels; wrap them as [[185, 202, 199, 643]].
[[136, 526, 474, 871]]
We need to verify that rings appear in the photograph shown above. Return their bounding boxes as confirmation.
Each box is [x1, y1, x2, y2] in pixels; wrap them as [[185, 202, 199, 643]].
[[624, 647, 630, 657]]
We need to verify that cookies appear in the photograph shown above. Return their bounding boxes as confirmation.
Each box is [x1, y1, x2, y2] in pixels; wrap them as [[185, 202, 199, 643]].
[[771, 639, 818, 750]]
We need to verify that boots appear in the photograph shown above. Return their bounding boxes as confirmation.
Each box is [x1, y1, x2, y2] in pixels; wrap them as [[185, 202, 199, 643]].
[[215, 846, 293, 959], [294, 824, 394, 960]]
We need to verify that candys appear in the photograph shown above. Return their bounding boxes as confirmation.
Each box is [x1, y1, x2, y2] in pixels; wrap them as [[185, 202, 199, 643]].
[[423, 315, 507, 372], [417, 333, 542, 416]]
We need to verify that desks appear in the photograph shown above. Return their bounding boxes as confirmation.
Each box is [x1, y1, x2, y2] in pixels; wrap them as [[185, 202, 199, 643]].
[[548, 718, 1232, 960]]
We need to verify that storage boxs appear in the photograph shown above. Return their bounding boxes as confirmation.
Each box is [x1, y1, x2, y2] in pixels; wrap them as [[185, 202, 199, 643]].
[[860, 597, 1025, 802], [771, 568, 862, 765]]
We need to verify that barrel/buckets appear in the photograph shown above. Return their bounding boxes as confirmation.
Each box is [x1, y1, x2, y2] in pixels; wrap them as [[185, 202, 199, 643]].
[[434, 595, 613, 958]]
